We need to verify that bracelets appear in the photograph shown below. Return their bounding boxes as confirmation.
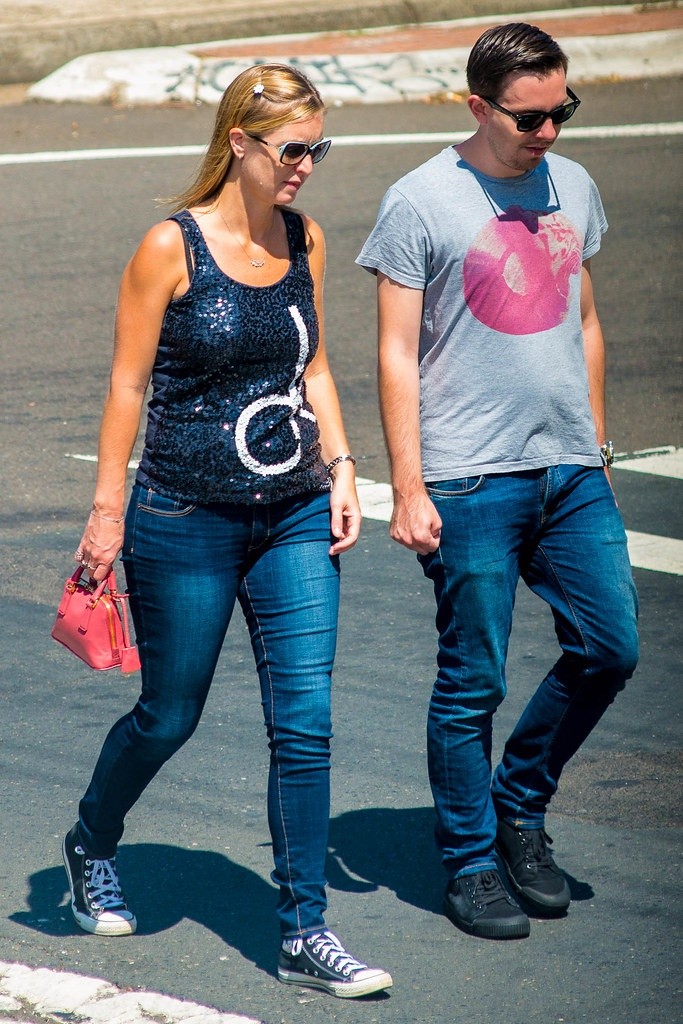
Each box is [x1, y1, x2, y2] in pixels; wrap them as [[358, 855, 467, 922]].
[[326, 455, 356, 472], [602, 440, 614, 464]]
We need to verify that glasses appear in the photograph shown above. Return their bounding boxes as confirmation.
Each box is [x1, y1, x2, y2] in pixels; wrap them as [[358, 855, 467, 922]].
[[244, 131, 331, 168], [483, 86, 581, 132]]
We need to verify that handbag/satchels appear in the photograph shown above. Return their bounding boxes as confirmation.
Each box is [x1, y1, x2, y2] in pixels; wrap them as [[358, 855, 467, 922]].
[[52, 564, 142, 674]]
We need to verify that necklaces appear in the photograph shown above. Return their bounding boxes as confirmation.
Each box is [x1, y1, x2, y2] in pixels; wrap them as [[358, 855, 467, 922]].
[[219, 209, 275, 267]]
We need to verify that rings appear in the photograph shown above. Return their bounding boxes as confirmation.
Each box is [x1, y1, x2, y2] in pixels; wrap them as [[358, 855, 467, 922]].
[[74, 550, 84, 560], [88, 564, 96, 570], [81, 560, 88, 569]]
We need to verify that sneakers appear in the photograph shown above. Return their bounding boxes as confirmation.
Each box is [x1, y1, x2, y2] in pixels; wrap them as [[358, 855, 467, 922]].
[[493, 810, 570, 916], [63, 821, 137, 936], [443, 867, 529, 938], [276, 929, 393, 997]]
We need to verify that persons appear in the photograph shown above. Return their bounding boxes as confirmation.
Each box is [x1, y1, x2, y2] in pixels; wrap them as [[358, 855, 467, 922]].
[[356, 24, 641, 935], [61, 64, 392, 997]]
